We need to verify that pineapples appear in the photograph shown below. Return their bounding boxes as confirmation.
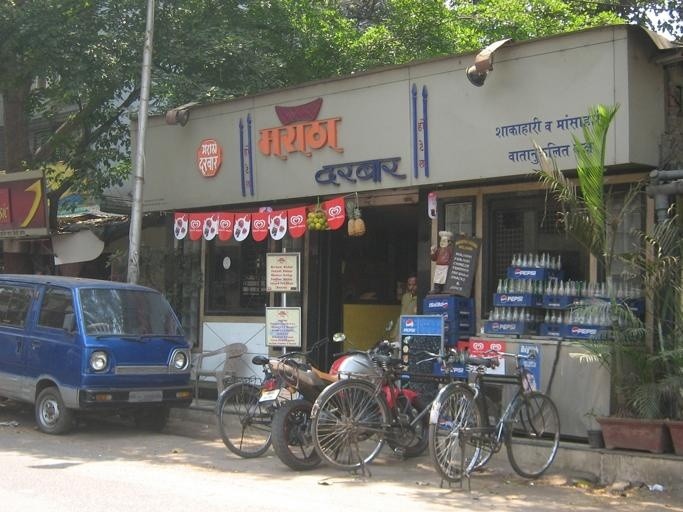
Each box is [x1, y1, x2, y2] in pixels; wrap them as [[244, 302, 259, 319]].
[[353, 208, 365, 237], [347, 201, 355, 237]]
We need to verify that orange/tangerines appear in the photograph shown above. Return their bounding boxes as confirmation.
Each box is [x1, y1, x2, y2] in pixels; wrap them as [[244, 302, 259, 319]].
[[307, 211, 328, 231]]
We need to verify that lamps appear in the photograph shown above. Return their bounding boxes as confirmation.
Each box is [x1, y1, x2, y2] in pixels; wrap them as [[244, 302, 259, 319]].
[[166, 101, 200, 126], [466, 38, 512, 86]]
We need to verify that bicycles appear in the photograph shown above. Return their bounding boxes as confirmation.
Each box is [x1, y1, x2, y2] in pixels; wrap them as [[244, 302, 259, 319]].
[[310, 347, 562, 490], [216, 336, 331, 459]]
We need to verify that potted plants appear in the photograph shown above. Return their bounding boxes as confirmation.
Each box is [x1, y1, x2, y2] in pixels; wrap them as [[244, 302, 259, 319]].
[[584, 410, 604, 448], [529, 104, 666, 455], [572, 213, 683, 457]]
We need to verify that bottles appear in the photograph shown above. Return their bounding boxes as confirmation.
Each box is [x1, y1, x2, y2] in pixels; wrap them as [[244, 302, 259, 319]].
[[488, 251, 636, 327]]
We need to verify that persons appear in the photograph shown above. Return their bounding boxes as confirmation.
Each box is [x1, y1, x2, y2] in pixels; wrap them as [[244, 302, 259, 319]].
[[388, 276, 417, 339], [429, 231, 454, 295]]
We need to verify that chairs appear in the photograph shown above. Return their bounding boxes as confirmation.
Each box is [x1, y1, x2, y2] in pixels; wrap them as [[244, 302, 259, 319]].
[[190, 343, 247, 401]]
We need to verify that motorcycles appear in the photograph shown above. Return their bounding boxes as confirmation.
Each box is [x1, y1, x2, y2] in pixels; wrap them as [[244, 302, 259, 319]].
[[252, 320, 429, 472]]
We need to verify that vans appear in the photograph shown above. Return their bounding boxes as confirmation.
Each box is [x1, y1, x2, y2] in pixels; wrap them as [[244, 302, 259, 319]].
[[0, 272, 194, 435]]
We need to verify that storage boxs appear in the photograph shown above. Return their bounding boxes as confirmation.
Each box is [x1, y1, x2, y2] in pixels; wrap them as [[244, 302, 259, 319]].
[[424, 266, 645, 379]]
